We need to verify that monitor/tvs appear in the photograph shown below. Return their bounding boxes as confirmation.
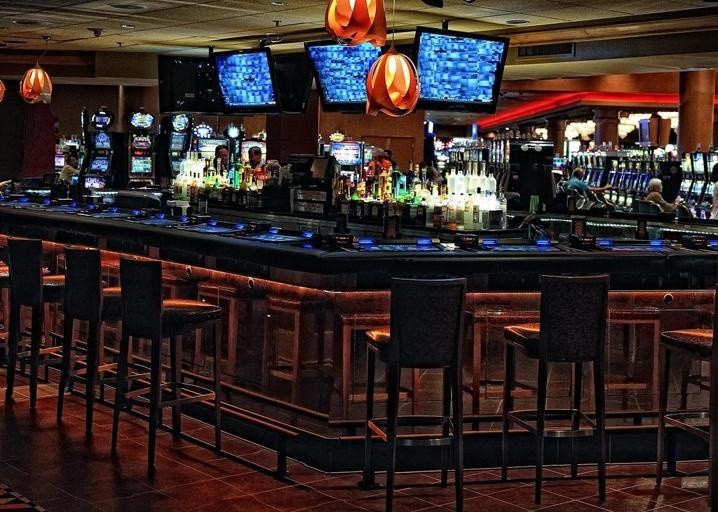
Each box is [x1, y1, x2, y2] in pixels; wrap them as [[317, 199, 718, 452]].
[[410, 26, 510, 114], [584, 169, 651, 191], [54, 154, 66, 167], [196, 136, 230, 160], [303, 39, 388, 114], [84, 155, 184, 188], [680, 176, 715, 195], [239, 139, 267, 166], [208, 46, 282, 114], [156, 53, 224, 116], [329, 140, 363, 166], [271, 52, 315, 116]]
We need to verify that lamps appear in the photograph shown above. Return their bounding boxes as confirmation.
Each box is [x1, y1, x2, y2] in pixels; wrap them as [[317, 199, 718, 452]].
[[20, 61, 52, 104], [325, 0, 386, 49], [365, 0, 420, 118]]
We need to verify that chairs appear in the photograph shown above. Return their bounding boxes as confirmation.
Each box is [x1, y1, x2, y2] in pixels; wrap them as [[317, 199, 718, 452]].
[[110, 255, 221, 471], [6, 234, 84, 413], [354, 275, 468, 507], [57, 245, 150, 436], [655, 327, 713, 489], [500, 274, 609, 502]]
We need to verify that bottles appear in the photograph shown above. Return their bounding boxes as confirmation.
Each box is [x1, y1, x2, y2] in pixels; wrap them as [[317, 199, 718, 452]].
[[336, 161, 508, 230], [170, 152, 294, 213]]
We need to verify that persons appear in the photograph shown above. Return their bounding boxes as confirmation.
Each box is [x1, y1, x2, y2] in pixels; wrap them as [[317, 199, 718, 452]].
[[567, 167, 612, 199], [247, 146, 263, 170], [213, 145, 233, 176], [383, 149, 397, 171], [368, 148, 392, 176], [709, 162, 718, 219], [57, 155, 88, 185], [646, 178, 682, 212]]
